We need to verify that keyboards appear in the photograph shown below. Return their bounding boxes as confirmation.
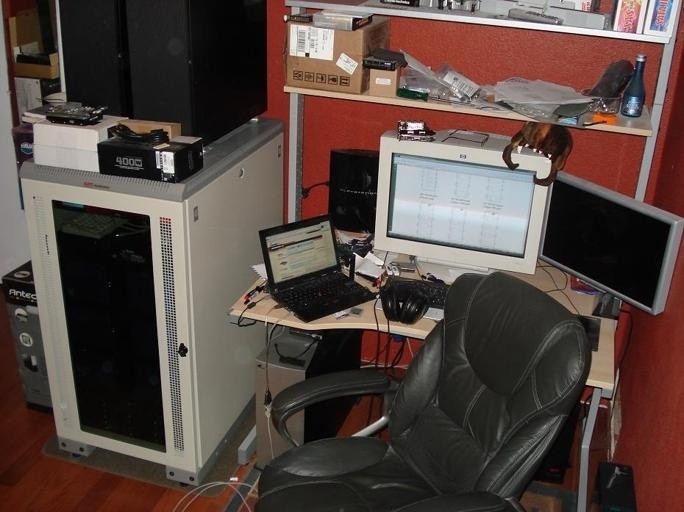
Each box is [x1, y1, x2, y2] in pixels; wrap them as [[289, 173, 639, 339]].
[[413, 281, 454, 310]]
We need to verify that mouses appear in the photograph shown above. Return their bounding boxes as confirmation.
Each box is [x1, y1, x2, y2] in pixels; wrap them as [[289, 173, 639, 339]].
[[574, 313, 589, 328]]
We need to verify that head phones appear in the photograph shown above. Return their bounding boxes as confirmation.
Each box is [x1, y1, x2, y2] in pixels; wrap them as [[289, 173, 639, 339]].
[[375, 283, 431, 325]]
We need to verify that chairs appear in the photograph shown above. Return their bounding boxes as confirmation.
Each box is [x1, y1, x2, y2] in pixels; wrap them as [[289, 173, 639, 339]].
[[253, 271, 592, 511]]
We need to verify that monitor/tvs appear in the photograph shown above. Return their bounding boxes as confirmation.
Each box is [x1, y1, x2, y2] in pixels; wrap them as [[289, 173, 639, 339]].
[[536, 168, 684, 319], [370, 125, 552, 282]]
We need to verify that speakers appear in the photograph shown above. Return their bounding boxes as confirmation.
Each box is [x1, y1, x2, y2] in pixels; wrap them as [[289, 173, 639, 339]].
[[326, 146, 380, 233]]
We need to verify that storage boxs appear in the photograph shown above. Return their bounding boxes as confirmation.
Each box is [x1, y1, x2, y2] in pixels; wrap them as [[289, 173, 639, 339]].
[[1, 260, 54, 415], [11, 77, 61, 126], [282, 15, 389, 94], [32, 116, 125, 174]]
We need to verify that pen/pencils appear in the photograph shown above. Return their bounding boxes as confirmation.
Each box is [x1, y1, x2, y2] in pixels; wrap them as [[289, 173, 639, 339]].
[[245, 286, 259, 299], [244, 285, 264, 304]]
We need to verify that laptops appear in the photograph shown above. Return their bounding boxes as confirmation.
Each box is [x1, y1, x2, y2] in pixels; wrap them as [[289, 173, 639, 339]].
[[258, 214, 376, 324]]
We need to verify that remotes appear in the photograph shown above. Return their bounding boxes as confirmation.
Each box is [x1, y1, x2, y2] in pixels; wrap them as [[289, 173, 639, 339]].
[[509, 8, 563, 25]]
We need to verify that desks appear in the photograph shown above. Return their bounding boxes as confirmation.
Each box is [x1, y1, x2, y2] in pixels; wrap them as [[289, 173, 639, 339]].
[[227, 249, 623, 512]]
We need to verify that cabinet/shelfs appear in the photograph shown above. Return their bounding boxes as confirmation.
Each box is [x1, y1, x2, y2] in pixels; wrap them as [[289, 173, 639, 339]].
[[284, 0, 682, 315]]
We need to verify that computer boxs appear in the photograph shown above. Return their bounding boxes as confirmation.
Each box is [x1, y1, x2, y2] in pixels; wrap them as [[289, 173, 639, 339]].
[[255, 329, 352, 471]]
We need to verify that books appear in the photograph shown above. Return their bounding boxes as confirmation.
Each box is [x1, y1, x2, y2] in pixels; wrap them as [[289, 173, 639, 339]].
[[21, 105, 56, 124], [15, 52, 59, 79]]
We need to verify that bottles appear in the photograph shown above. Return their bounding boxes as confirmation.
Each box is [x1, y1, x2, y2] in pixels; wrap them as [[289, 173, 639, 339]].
[[620, 54, 647, 118]]
